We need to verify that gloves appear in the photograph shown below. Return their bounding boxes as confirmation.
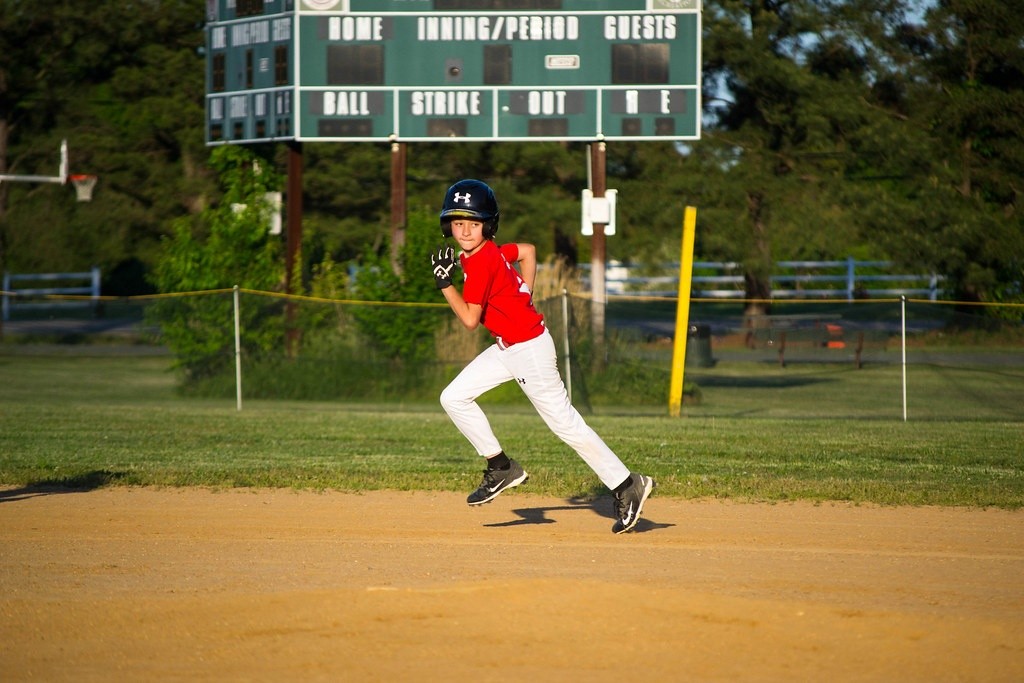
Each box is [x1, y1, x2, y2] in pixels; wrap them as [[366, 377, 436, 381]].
[[428, 243, 457, 290]]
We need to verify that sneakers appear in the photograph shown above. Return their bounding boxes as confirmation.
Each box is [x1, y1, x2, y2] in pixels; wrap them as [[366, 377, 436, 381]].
[[467, 458, 529, 505], [612, 472, 656, 534]]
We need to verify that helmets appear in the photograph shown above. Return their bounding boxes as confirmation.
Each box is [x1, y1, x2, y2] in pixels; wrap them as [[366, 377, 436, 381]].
[[439, 179, 499, 239]]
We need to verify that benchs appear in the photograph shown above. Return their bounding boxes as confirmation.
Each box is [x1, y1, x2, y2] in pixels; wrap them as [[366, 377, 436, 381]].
[[0, 268, 102, 320]]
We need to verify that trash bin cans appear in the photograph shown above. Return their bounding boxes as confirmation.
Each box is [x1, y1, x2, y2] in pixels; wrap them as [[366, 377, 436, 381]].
[[686, 325, 713, 368]]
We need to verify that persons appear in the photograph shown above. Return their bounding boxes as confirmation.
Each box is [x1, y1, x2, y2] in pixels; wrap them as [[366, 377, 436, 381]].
[[431, 179, 655, 536], [853, 282, 870, 299]]
